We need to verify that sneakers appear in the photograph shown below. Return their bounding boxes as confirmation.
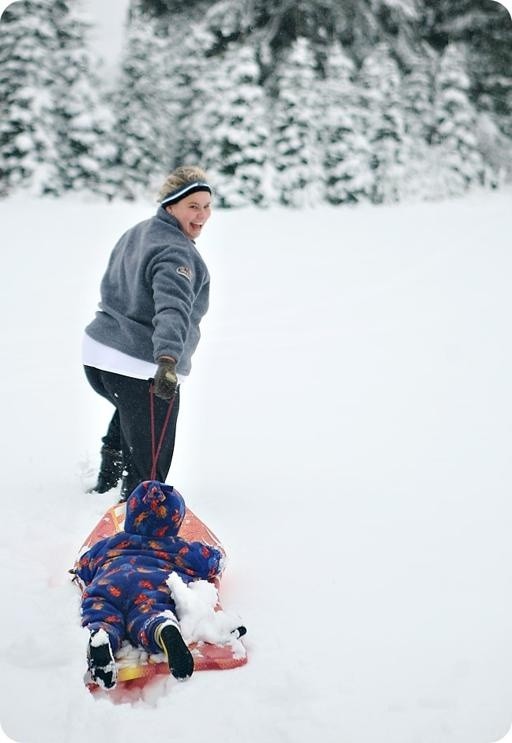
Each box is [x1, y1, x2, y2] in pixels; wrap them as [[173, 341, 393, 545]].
[[88, 630, 118, 691], [157, 622, 195, 682]]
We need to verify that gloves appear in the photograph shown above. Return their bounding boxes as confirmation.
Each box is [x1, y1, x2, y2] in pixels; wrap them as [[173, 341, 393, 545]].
[[152, 357, 180, 403]]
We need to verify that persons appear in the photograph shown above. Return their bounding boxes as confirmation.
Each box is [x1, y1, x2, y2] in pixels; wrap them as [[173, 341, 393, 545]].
[[80, 166, 213, 504], [74, 480, 226, 693]]
